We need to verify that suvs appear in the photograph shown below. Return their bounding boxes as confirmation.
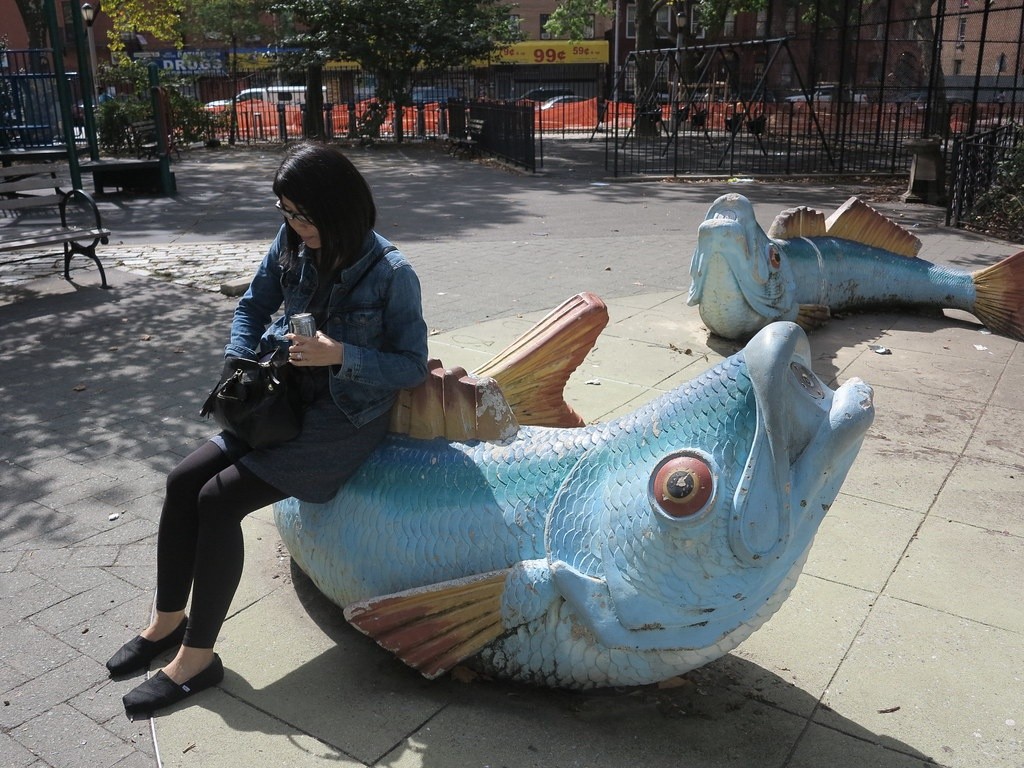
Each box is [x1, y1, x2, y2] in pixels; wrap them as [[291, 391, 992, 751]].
[[505, 86, 574, 103]]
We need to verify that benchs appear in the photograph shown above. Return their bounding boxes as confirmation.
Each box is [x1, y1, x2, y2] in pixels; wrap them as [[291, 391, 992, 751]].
[[0, 164, 113, 289], [76, 160, 160, 195], [445, 119, 484, 160], [131, 118, 182, 160]]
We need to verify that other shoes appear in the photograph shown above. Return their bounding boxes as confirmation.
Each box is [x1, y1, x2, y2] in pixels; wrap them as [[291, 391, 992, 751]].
[[122, 651, 223, 714], [106, 613, 189, 676]]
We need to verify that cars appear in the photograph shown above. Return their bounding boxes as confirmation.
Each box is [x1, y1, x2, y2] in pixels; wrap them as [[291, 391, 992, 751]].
[[785, 86, 870, 114], [534, 96, 588, 110]]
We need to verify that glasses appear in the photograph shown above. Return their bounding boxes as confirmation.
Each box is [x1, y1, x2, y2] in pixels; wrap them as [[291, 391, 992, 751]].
[[275, 199, 314, 226]]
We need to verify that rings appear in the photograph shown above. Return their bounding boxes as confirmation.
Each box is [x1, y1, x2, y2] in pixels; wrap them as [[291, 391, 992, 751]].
[[296, 352, 302, 360]]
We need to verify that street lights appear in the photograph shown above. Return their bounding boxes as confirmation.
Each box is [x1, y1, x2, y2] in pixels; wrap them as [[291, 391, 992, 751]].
[[81, 3, 99, 108]]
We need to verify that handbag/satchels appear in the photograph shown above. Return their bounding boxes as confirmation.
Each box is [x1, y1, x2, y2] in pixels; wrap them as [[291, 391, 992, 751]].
[[199, 357, 308, 444]]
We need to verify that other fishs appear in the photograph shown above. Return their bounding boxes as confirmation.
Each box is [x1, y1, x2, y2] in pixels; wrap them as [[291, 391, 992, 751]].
[[272, 291, 873, 690], [685, 192, 1024, 342]]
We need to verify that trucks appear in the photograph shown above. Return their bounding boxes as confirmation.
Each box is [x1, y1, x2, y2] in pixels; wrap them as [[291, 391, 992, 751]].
[[204, 85, 327, 108]]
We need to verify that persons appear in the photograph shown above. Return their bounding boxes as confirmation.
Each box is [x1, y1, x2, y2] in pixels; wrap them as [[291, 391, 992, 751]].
[[106, 142, 428, 715]]
